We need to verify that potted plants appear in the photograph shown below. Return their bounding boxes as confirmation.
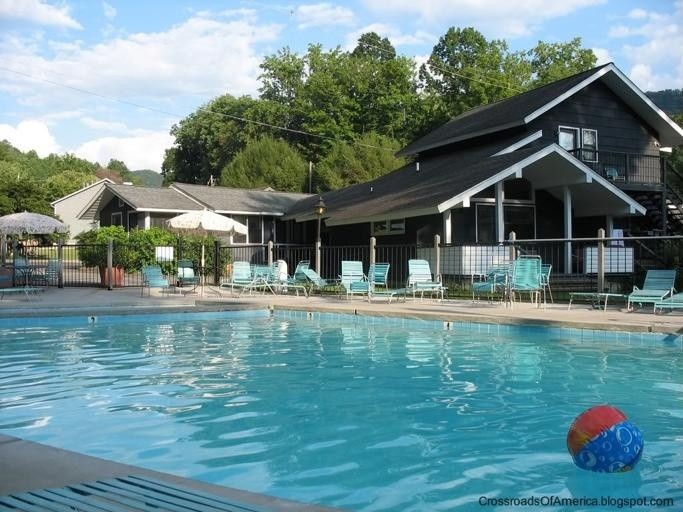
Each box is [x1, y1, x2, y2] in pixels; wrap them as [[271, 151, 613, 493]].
[[74, 225, 155, 288]]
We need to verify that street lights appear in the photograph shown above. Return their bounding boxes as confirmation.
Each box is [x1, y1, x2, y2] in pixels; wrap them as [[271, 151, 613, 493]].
[[313, 196, 327, 277]]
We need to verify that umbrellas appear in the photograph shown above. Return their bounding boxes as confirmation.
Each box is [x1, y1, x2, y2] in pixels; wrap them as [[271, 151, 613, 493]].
[[0, 209, 70, 287], [165, 206, 249, 293]]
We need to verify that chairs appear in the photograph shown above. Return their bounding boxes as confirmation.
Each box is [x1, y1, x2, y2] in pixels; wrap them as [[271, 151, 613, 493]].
[[0, 255, 683, 316], [0, 473, 283, 512]]
